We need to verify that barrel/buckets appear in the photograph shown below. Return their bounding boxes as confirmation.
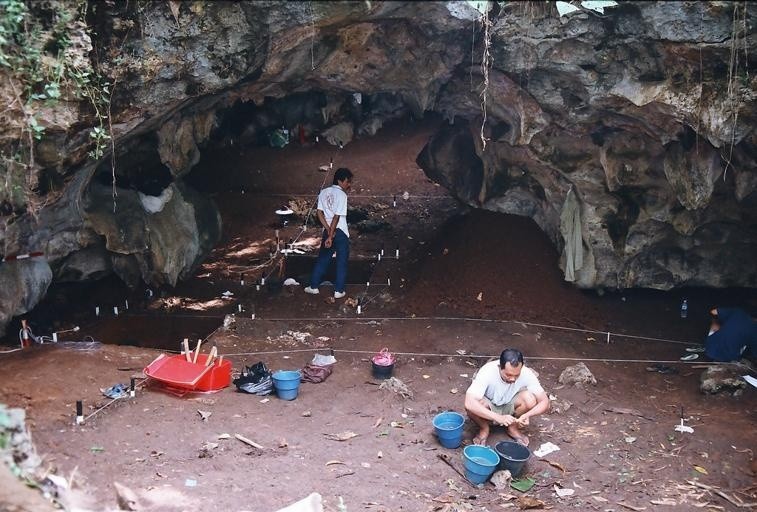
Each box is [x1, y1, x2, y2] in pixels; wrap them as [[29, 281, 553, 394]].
[[271, 372, 302, 400], [371, 358, 394, 379], [463, 444, 500, 485], [433, 412, 465, 450], [494, 440, 532, 478]]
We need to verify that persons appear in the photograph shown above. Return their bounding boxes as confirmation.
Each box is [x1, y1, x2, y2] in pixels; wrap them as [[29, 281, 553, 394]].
[[303, 168, 354, 298], [704, 305, 757, 364], [465, 349, 550, 447]]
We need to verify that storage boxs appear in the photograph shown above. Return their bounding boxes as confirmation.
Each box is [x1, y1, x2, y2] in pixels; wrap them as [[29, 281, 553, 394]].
[[143, 350, 232, 394]]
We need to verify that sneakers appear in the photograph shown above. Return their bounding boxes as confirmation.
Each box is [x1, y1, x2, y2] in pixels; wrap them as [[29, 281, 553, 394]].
[[334, 291, 346, 299], [304, 286, 319, 295]]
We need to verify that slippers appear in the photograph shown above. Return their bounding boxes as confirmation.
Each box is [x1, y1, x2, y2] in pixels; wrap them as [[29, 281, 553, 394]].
[[507, 430, 529, 447], [473, 434, 487, 445]]
